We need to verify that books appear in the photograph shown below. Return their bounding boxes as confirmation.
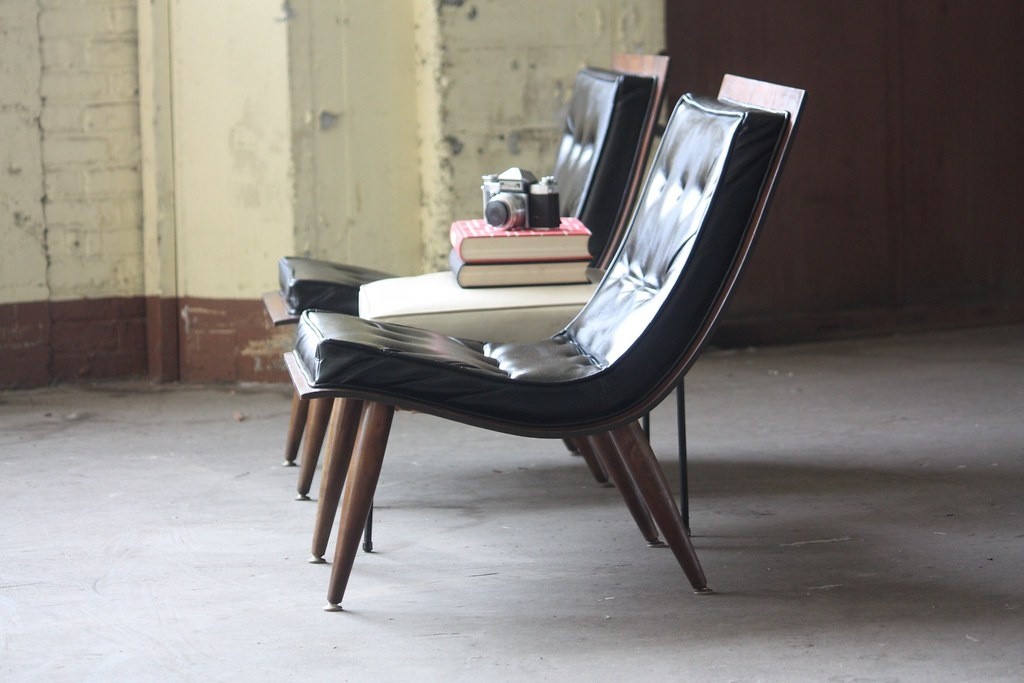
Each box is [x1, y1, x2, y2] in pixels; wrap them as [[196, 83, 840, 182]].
[[448, 217, 594, 288]]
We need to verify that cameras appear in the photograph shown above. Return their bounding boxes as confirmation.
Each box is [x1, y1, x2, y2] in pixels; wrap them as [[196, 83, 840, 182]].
[[480, 167, 560, 230]]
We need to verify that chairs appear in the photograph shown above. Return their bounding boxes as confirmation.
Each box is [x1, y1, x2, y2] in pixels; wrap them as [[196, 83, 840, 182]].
[[263, 50, 808, 612]]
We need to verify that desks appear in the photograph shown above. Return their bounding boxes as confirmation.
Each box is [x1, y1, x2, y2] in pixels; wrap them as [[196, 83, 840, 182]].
[[357, 267, 689, 548]]
